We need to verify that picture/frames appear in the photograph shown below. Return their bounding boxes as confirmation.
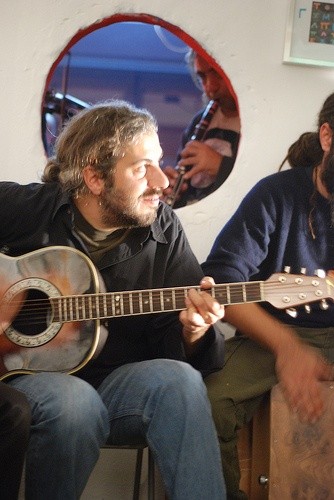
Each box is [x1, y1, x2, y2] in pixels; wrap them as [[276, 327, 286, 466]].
[[281, 1, 334, 69]]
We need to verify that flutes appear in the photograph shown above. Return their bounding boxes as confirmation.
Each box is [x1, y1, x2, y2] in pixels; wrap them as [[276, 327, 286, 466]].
[[163, 98, 219, 209]]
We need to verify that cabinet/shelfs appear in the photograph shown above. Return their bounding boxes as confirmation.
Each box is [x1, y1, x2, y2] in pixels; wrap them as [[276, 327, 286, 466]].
[[233, 393, 271, 500]]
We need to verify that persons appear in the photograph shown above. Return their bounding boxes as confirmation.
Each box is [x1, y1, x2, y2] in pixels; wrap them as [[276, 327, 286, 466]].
[[159, 47, 240, 209], [202, 93, 334, 499], [0, 100, 227, 500]]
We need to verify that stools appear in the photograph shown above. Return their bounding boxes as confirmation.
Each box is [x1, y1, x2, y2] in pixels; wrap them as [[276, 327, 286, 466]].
[[16, 433, 159, 499]]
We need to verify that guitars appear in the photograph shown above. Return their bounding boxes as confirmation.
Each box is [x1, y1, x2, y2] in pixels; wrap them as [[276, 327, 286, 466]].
[[0, 245, 334, 380]]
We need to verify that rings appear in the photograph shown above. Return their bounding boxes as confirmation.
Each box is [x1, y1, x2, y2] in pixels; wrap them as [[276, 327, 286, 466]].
[[191, 157, 194, 165]]
